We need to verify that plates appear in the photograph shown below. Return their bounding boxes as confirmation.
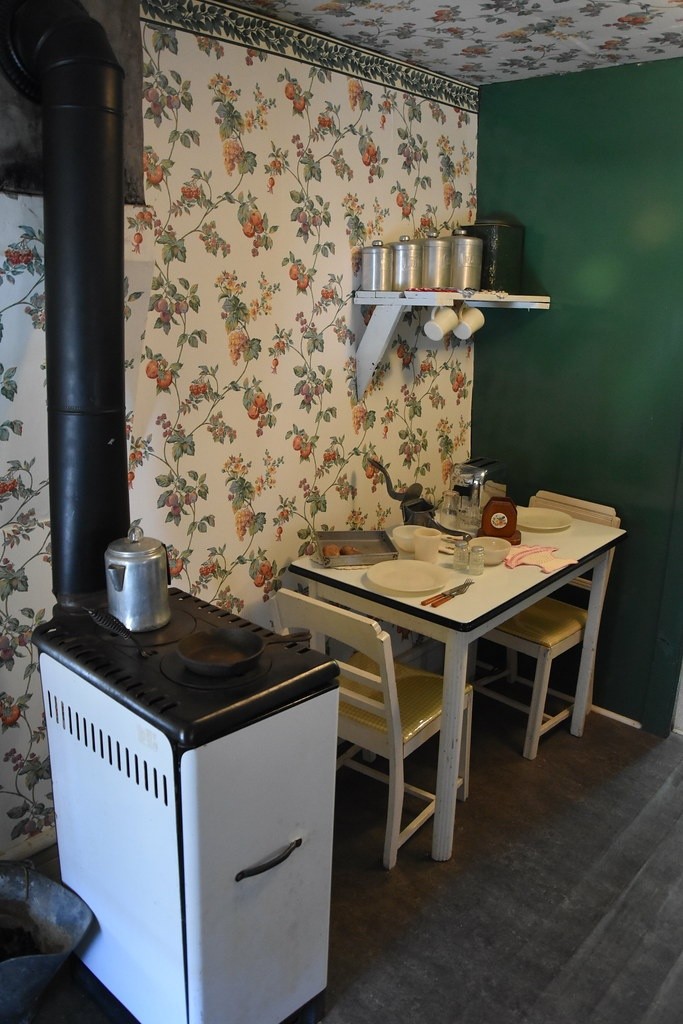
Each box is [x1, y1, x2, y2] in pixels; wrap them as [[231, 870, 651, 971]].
[[368, 559, 448, 591], [517, 508, 573, 531]]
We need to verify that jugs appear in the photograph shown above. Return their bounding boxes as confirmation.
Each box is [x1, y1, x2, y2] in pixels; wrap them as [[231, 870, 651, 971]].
[[105, 526, 173, 633]]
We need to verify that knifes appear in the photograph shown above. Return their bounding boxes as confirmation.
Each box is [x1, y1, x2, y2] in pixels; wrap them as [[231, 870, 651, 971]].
[[422, 581, 475, 604]]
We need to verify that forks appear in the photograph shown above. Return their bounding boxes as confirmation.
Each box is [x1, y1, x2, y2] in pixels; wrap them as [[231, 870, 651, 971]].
[[432, 578, 472, 607]]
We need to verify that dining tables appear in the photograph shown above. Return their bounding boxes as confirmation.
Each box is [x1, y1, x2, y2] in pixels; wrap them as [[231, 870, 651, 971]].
[[290, 505, 625, 861]]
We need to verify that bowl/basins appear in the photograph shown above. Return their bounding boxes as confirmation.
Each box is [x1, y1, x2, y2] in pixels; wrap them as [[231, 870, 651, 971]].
[[394, 524, 426, 552], [469, 537, 511, 565]]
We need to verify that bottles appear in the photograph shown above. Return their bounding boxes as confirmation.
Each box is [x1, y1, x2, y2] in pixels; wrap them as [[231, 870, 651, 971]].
[[470, 546, 485, 576], [453, 542, 468, 568]]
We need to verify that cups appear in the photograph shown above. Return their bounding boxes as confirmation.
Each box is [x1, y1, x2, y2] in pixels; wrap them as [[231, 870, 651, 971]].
[[440, 491, 460, 528], [453, 304, 485, 340], [414, 529, 441, 565], [461, 497, 480, 531], [424, 306, 459, 343]]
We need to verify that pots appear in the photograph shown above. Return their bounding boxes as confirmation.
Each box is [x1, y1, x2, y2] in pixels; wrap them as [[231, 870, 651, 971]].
[[179, 627, 311, 676]]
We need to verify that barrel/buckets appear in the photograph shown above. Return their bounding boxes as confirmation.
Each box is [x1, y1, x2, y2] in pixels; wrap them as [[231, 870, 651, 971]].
[[443, 229, 483, 291], [389, 236, 422, 289], [361, 241, 393, 291], [422, 233, 450, 288]]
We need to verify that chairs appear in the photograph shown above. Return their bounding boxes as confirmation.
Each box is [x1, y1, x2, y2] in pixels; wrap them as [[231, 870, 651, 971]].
[[466, 491, 620, 760], [267, 587, 473, 868]]
